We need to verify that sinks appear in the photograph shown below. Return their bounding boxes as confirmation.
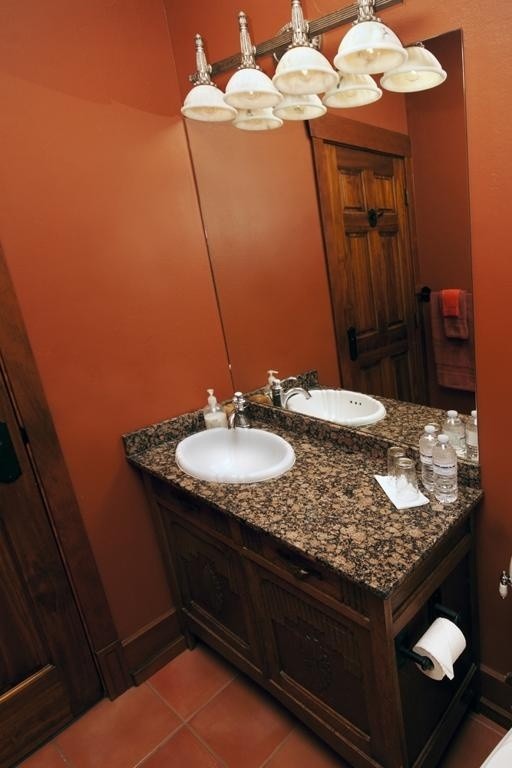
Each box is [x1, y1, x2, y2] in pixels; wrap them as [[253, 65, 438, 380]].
[[289, 387, 386, 427], [177, 429, 295, 487]]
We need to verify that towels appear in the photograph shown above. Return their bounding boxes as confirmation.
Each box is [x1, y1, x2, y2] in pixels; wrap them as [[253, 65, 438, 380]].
[[441, 289, 471, 340], [439, 286, 463, 317], [427, 285, 476, 392]]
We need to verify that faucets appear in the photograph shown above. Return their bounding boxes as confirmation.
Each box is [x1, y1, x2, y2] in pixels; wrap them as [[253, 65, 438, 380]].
[[283, 385, 311, 405], [228, 406, 243, 432]]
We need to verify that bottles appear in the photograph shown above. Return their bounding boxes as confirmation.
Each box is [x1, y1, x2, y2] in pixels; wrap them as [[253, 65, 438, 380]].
[[466, 409, 479, 461], [418, 424, 441, 495], [441, 409, 468, 459], [431, 433, 458, 503]]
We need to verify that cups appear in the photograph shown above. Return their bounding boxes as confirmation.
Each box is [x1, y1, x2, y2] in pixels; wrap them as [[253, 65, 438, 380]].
[[391, 457, 419, 502], [386, 446, 408, 488], [427, 422, 441, 444]]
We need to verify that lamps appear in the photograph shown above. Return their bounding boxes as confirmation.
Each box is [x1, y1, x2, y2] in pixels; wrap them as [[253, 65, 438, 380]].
[[231, 40, 451, 134], [178, 1, 409, 126]]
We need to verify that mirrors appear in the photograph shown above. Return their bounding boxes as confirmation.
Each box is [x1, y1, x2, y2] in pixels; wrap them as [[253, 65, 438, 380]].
[[175, 25, 485, 473]]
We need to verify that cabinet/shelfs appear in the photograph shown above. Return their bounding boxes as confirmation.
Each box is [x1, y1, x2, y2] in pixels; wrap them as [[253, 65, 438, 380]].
[[137, 466, 486, 766]]
